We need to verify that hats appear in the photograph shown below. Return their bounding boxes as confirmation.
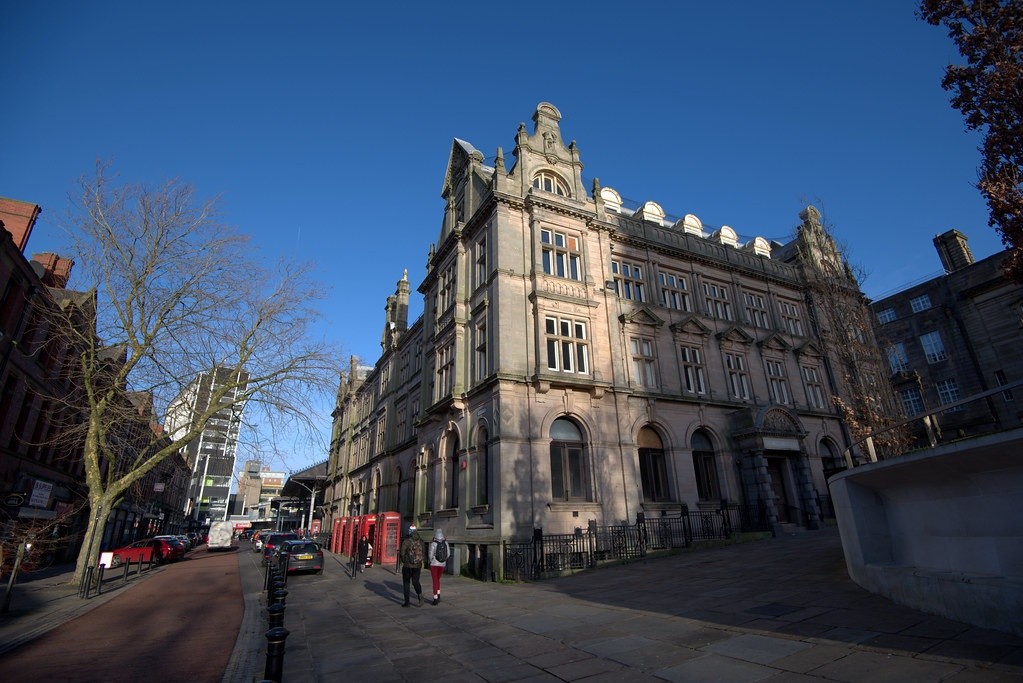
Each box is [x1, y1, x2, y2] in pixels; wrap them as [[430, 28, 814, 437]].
[[434, 528, 443, 539]]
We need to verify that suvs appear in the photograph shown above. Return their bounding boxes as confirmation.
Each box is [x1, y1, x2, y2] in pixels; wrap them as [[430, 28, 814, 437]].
[[261, 532, 301, 558]]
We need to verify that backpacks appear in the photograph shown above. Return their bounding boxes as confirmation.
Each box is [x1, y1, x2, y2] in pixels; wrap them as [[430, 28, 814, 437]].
[[407, 539, 423, 564], [435, 540, 448, 562]]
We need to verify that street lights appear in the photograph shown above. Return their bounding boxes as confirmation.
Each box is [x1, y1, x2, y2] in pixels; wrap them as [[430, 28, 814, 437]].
[[0, 542, 33, 607], [289, 477, 317, 539]]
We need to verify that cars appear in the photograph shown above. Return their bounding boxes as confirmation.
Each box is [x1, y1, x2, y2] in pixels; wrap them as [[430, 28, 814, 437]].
[[250, 528, 276, 552], [111, 538, 177, 570], [153, 532, 203, 559], [271, 539, 324, 574], [239, 530, 257, 540]]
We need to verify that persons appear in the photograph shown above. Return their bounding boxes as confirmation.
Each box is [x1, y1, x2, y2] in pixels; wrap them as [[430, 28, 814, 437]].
[[409, 520, 416, 529], [429, 529, 450, 605], [251, 531, 260, 553], [358, 535, 368, 573], [400, 523, 424, 608], [289, 527, 310, 536], [239, 531, 242, 540]]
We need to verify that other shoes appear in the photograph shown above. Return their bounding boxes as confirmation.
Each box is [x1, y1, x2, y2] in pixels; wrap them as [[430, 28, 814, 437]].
[[438, 594, 440, 599], [433, 599, 439, 606], [418, 595, 424, 604], [402, 602, 410, 607]]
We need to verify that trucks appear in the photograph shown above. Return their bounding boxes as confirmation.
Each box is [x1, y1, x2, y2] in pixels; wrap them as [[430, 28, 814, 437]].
[[206, 521, 234, 551]]
